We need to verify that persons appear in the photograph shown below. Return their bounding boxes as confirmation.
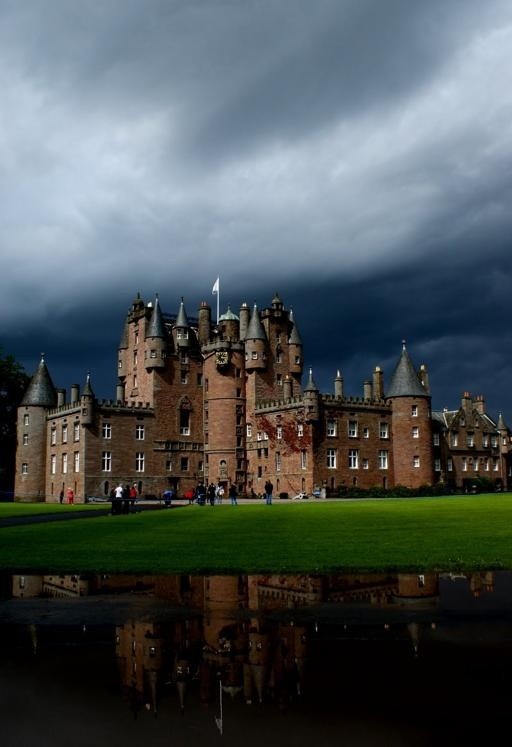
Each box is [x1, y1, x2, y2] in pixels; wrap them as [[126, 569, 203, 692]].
[[265, 479, 273, 504], [216, 482, 224, 503], [229, 483, 238, 506], [109, 482, 139, 514], [59, 487, 75, 505], [191, 482, 216, 506]]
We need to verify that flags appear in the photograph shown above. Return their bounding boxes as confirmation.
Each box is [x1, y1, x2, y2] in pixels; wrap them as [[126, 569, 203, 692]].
[[214, 715, 224, 736], [212, 277, 220, 295]]
